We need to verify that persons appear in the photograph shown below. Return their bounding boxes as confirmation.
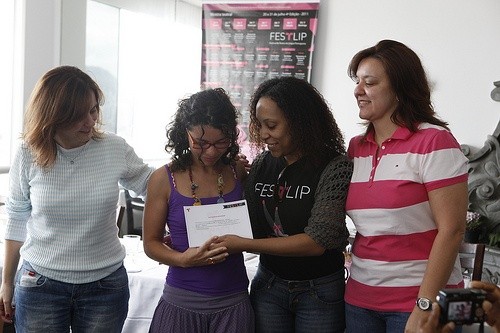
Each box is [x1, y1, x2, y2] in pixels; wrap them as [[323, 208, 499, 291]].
[[207, 75, 353, 333], [449, 304, 466, 320], [0, 66, 251, 333], [142, 87, 256, 333], [343, 40, 469, 333], [437, 280, 500, 333]]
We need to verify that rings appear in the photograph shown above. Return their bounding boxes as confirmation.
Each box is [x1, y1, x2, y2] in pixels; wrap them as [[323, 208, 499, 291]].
[[208, 258, 213, 262], [212, 261, 214, 264]]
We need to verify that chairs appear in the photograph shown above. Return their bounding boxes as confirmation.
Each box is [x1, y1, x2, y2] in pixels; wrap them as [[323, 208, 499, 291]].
[[116, 190, 146, 237]]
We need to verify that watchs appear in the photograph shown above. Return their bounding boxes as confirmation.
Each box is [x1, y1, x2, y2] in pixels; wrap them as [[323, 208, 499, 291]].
[[415, 297, 432, 312]]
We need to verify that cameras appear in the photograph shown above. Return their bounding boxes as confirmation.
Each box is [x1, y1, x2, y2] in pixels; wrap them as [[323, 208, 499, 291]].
[[436, 288, 489, 325]]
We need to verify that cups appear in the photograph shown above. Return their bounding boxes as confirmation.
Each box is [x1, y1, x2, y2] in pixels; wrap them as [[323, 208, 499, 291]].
[[122, 234, 140, 257]]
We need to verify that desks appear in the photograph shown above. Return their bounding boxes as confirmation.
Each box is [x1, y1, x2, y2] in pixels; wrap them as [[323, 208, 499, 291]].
[[117, 236, 260, 333]]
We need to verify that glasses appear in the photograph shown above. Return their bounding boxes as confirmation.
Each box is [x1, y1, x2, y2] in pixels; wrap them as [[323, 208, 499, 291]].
[[185, 128, 234, 149]]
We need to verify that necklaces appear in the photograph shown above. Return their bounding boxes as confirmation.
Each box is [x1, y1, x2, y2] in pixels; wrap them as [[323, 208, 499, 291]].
[[59, 146, 85, 164], [188, 166, 225, 205]]
